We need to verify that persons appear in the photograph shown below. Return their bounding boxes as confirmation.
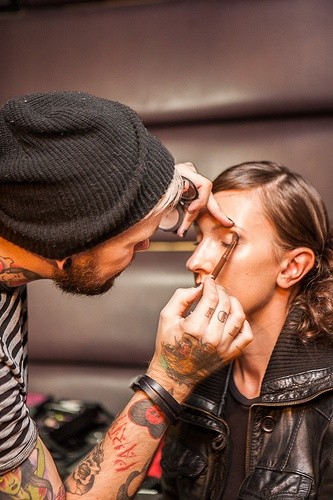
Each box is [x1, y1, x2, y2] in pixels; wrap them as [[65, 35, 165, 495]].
[[1, 91, 253, 499], [160, 160, 332, 498]]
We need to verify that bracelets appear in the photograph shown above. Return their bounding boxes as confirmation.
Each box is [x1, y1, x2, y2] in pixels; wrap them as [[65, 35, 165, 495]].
[[130, 374, 184, 427]]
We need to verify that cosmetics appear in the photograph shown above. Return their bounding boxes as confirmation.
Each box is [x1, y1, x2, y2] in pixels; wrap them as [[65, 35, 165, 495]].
[[157, 176, 198, 232]]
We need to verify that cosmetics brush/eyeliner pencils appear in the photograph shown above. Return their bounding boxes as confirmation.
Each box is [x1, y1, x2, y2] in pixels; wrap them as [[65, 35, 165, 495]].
[[185, 232, 239, 318]]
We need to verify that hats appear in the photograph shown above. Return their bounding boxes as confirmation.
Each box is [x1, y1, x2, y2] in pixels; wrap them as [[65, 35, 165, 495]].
[[0, 92, 176, 259]]
